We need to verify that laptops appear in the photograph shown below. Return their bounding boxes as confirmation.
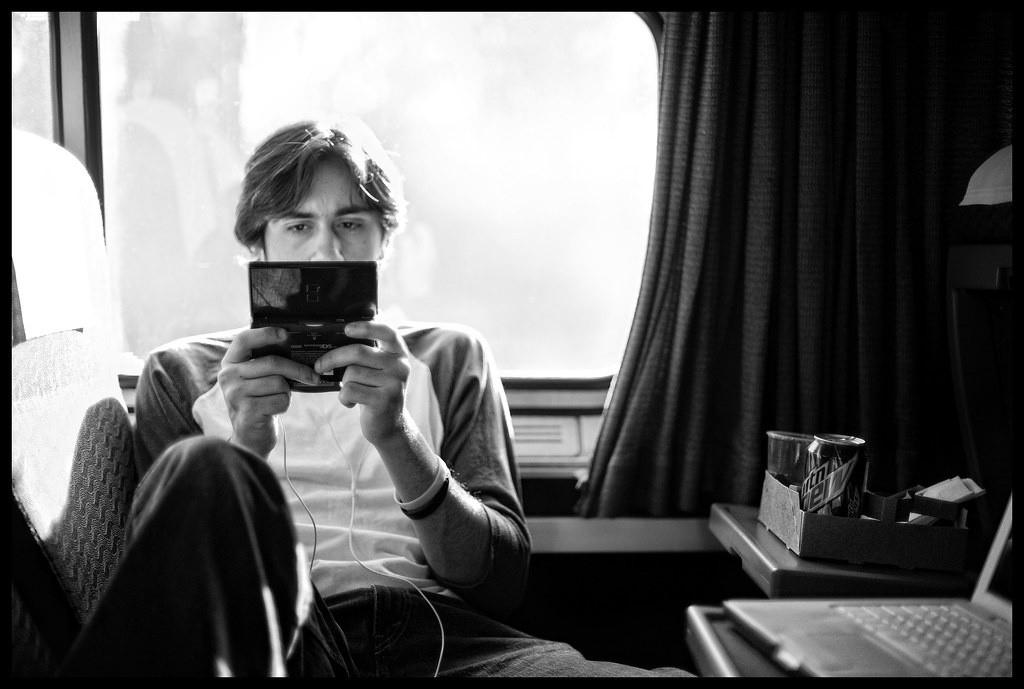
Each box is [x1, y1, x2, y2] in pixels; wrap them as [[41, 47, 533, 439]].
[[724, 487, 1013, 676]]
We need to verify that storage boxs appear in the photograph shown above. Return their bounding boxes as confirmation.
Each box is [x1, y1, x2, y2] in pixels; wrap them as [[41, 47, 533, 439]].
[[758, 473, 988, 570]]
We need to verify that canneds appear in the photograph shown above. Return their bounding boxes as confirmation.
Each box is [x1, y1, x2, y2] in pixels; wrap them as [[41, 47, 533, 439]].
[[801, 434, 869, 518]]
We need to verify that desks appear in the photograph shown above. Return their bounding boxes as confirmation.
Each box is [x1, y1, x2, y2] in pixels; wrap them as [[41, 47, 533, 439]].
[[709, 500, 974, 600], [685, 604, 806, 678]]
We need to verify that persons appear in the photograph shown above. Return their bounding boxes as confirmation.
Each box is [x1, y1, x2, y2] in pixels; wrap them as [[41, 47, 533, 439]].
[[49, 124, 700, 678]]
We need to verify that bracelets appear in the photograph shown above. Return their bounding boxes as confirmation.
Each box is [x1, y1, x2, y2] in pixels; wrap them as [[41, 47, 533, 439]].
[[400, 457, 454, 520]]
